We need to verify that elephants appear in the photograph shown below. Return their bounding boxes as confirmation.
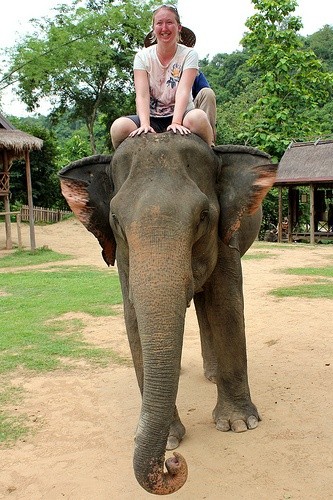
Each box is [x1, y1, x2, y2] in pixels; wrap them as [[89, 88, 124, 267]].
[[57, 129, 280, 496]]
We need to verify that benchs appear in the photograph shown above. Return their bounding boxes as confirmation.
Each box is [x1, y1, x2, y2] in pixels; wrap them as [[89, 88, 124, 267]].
[[291, 232, 333, 244]]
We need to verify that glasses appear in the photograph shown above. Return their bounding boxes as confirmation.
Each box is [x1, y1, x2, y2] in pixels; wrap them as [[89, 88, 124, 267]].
[[153, 4, 180, 28]]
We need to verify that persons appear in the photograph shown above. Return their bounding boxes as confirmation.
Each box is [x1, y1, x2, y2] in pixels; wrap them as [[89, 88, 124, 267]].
[[109, 4, 217, 151], [276, 216, 288, 234]]
